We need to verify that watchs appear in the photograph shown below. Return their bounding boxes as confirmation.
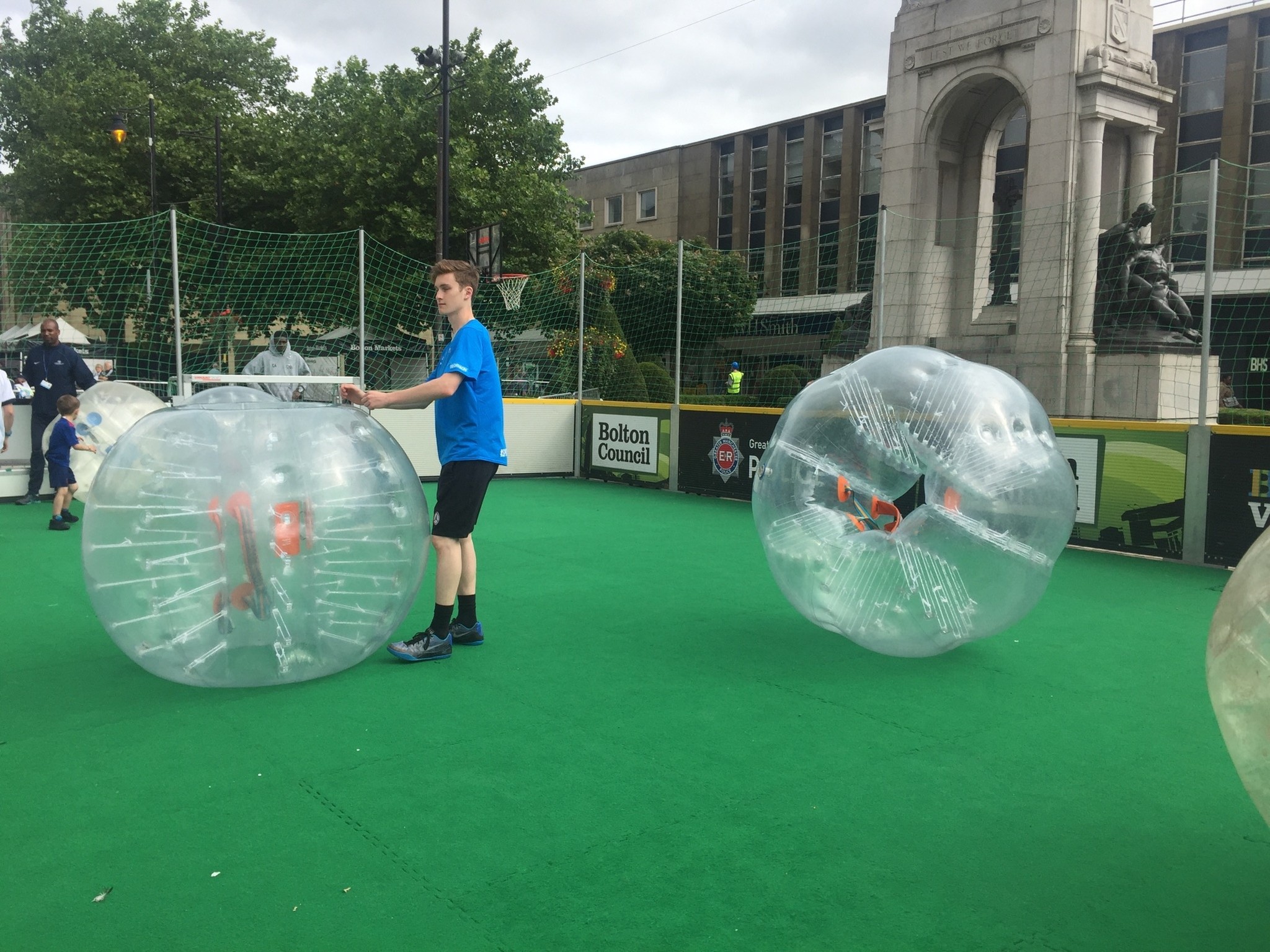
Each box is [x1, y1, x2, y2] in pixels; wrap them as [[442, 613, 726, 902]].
[[297, 386, 305, 393]]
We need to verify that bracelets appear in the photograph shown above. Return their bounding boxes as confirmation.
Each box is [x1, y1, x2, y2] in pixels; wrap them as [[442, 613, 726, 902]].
[[5, 431, 12, 436]]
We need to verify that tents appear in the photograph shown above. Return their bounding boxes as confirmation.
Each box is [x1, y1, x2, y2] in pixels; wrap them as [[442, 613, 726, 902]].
[[0, 316, 95, 375]]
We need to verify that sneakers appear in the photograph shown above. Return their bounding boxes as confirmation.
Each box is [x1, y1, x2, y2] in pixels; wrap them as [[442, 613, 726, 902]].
[[449, 617, 484, 646], [60, 510, 79, 523], [16, 492, 41, 505], [387, 628, 453, 661], [49, 515, 70, 530]]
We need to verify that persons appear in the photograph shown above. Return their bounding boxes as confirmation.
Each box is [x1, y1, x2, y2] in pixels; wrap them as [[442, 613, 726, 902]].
[[340, 260, 508, 661], [0, 362, 116, 530], [207, 331, 310, 402], [724, 362, 745, 395], [1220, 375, 1245, 408], [1100, 201, 1207, 344], [13, 320, 97, 506]]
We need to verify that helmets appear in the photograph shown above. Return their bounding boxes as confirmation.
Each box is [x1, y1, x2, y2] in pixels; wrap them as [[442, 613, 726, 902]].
[[732, 362, 738, 369]]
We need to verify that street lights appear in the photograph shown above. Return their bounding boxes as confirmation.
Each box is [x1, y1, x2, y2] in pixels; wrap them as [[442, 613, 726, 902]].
[[110, 92, 160, 277], [166, 116, 226, 314]]
